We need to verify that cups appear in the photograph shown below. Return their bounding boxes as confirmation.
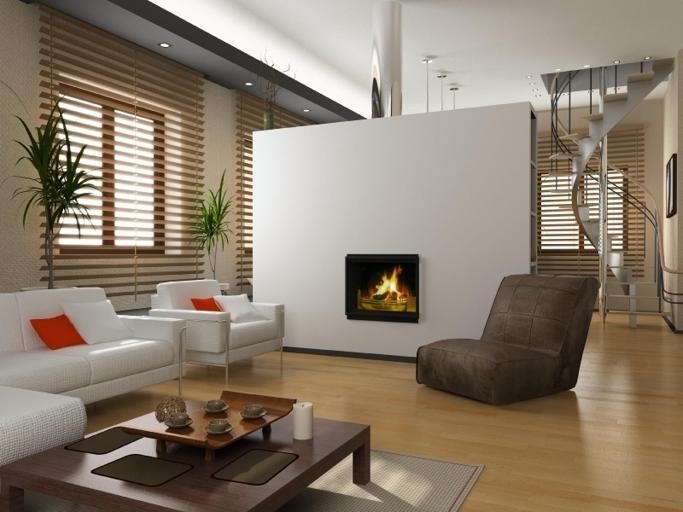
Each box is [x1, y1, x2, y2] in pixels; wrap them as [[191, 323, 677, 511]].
[[292, 402, 314, 441], [169, 414, 188, 424], [207, 400, 224, 410], [208, 418, 227, 433], [244, 404, 263, 417]]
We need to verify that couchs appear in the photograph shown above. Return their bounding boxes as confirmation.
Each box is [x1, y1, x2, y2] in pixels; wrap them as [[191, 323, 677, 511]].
[[0, 286, 191, 466]]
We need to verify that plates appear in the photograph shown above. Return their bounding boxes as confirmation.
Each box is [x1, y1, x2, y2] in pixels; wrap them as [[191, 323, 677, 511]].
[[203, 404, 229, 415], [239, 410, 267, 420], [165, 418, 192, 429], [203, 424, 233, 436]]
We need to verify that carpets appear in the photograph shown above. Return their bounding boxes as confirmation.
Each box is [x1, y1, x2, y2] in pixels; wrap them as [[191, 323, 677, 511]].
[[282, 443, 486, 511]]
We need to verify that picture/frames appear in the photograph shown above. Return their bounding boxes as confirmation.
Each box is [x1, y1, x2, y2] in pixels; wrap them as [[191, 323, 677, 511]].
[[665, 152, 677, 218]]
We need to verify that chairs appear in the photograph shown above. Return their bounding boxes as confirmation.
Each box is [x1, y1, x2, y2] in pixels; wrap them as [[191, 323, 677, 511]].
[[417, 271, 601, 404], [150, 279, 287, 388]]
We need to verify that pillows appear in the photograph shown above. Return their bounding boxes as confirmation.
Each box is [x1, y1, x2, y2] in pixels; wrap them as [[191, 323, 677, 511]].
[[29, 296, 132, 351], [189, 291, 264, 322]]
